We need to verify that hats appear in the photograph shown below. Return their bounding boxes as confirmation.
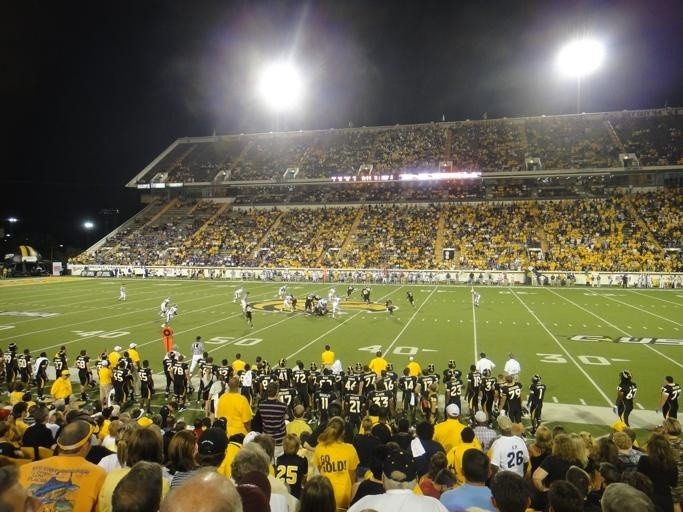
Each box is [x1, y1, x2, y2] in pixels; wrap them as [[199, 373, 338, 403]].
[[198, 428, 227, 455], [61, 370, 69, 376], [102, 343, 138, 366], [447, 404, 513, 432]]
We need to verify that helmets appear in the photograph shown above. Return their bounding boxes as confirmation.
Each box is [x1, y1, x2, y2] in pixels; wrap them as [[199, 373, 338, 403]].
[[403, 369, 409, 376], [347, 363, 361, 374], [311, 362, 317, 371], [532, 375, 542, 384], [8, 343, 18, 352], [429, 364, 434, 373], [387, 364, 392, 371], [279, 359, 287, 368], [483, 369, 491, 377], [622, 370, 633, 380], [448, 360, 456, 376]]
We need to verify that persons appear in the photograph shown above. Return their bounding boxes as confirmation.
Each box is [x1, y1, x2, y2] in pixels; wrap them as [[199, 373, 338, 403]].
[[240, 291, 250, 314], [161, 321, 173, 353], [245, 304, 254, 329], [69, 107, 683, 288], [2, 265, 8, 280], [470, 288, 482, 307], [2, 334, 682, 510], [277, 283, 371, 321], [158, 297, 170, 317], [7, 267, 12, 279], [385, 299, 396, 317], [406, 291, 416, 309], [159, 303, 178, 326], [117, 284, 128, 301], [232, 286, 243, 305]]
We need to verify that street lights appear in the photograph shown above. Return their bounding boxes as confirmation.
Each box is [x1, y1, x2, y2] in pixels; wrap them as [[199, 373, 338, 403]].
[[559, 40, 609, 112], [259, 73, 302, 134]]
